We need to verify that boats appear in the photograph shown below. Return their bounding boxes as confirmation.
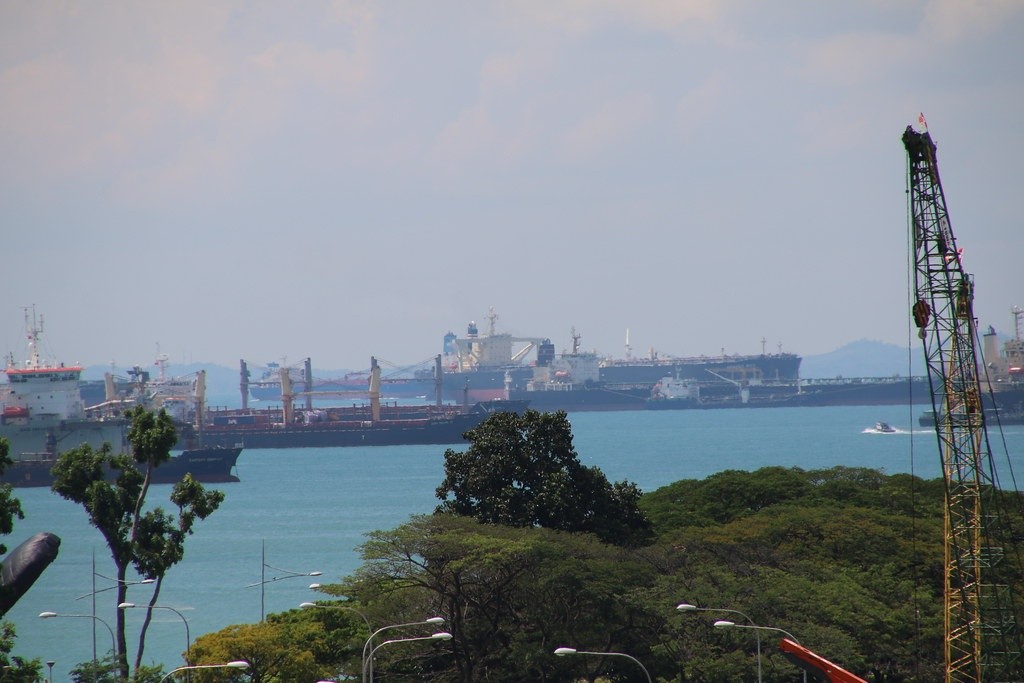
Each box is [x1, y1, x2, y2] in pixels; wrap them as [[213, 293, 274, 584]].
[[78, 341, 193, 407], [249, 354, 331, 402], [150, 357, 531, 450], [0, 303, 246, 488], [876, 421, 896, 432], [276, 304, 557, 401], [920, 367, 1024, 427]]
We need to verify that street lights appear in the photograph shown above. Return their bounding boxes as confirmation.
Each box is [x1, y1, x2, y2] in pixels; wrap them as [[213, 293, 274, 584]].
[[309, 582, 321, 591], [676, 603, 762, 683], [261, 539, 323, 625], [91, 546, 155, 666], [38, 612, 117, 683], [45, 661, 57, 683], [362, 633, 453, 683], [713, 620, 807, 683], [159, 661, 251, 683], [116, 602, 191, 683], [298, 602, 374, 683], [555, 648, 652, 683], [362, 617, 445, 683]]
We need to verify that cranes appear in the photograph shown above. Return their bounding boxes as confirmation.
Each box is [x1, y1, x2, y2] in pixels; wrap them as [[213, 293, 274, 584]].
[[901, 125, 1024, 683]]
